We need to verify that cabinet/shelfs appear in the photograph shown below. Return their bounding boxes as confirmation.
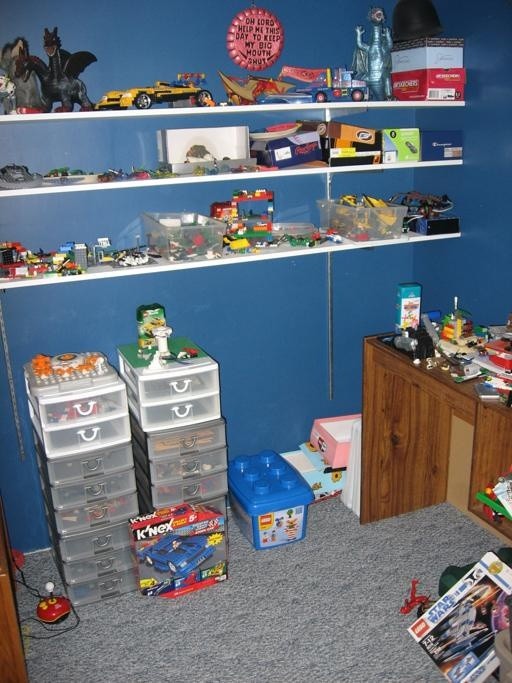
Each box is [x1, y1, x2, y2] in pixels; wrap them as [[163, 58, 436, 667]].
[[0, 100, 464, 290]]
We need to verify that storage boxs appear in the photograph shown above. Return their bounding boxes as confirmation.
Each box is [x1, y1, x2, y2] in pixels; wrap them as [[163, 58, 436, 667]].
[[229, 451, 315, 550], [280, 441, 344, 503], [310, 412, 359, 470], [23, 338, 232, 605]]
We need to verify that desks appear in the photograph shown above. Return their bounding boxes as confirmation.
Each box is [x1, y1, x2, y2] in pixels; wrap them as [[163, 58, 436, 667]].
[[359, 331, 512, 547]]
